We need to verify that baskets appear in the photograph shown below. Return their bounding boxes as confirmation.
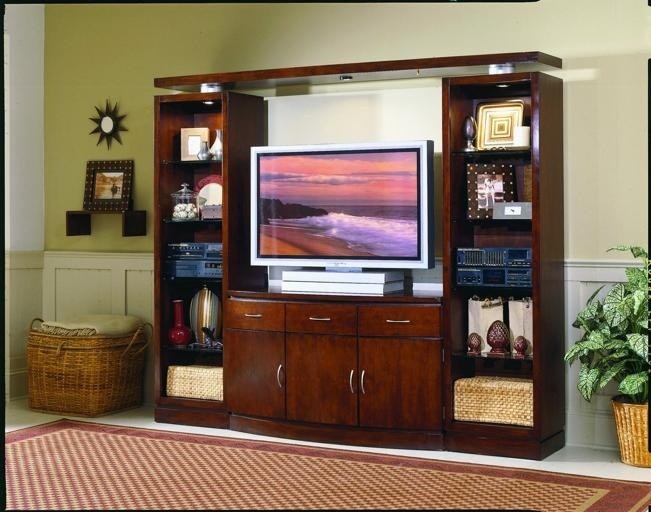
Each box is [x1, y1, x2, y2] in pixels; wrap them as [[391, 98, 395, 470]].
[[25, 318, 153, 418]]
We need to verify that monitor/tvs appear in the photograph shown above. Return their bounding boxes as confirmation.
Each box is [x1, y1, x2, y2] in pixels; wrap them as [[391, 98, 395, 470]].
[[251, 140, 433, 283]]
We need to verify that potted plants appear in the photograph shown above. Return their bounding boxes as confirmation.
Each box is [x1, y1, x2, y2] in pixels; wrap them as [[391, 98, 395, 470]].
[[564, 242, 651, 468]]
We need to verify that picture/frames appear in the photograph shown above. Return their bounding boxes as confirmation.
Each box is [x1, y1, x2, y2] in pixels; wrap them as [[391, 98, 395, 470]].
[[180, 127, 209, 161], [82, 160, 134, 213], [467, 162, 514, 218], [474, 98, 525, 148]]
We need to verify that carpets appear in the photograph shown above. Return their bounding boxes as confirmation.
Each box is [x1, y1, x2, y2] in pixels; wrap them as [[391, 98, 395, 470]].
[[4, 418, 651, 509]]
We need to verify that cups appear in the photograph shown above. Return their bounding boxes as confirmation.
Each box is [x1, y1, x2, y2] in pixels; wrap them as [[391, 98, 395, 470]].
[[512, 126, 530, 146]]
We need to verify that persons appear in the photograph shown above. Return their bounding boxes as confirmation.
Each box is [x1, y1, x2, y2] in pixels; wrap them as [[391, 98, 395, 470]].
[[484, 176, 496, 206]]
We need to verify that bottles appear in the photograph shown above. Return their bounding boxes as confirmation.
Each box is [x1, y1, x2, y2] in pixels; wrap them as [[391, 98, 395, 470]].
[[197, 128, 224, 161], [170, 183, 200, 221]]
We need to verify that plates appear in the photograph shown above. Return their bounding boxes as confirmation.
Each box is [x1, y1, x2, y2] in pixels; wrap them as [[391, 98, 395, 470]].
[[505, 146, 531, 151]]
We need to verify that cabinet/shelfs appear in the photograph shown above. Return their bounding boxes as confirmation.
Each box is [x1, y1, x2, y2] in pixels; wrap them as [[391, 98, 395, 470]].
[[446, 78, 536, 440], [159, 99, 221, 401], [224, 296, 448, 432]]
